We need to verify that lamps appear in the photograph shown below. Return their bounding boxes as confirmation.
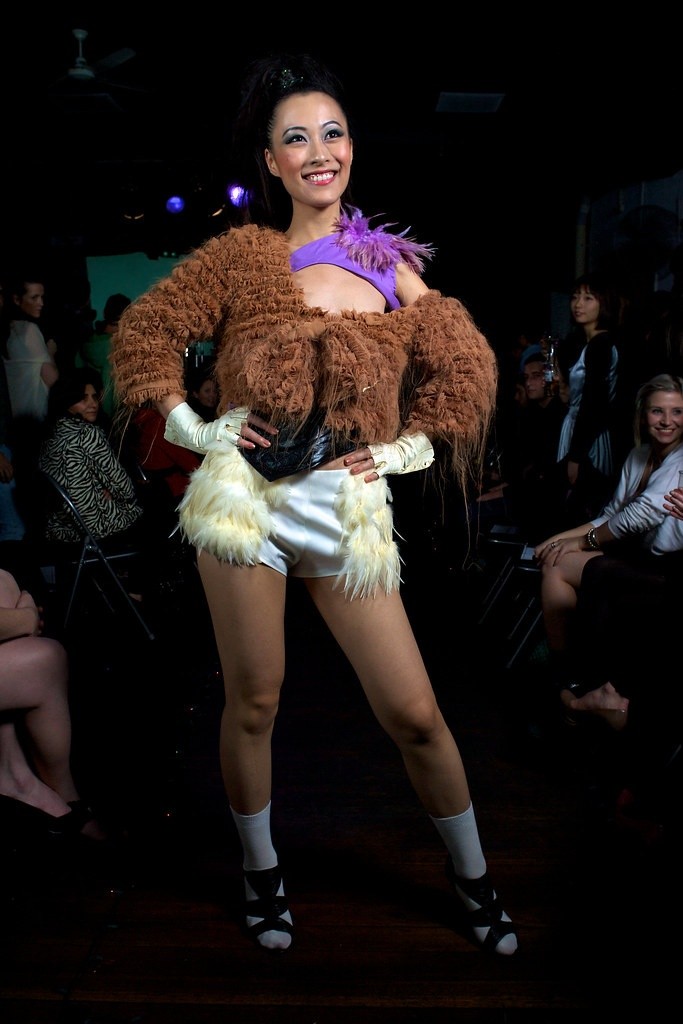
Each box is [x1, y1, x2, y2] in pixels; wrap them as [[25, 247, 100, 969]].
[[192, 181, 227, 219], [123, 187, 145, 221], [67, 29, 95, 81]]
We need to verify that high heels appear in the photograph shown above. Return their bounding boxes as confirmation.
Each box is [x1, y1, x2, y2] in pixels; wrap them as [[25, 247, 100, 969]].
[[240, 864, 296, 954], [444, 858, 521, 955]]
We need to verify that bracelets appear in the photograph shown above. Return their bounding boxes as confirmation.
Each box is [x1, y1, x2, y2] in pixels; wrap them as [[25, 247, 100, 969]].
[[588, 528, 601, 550]]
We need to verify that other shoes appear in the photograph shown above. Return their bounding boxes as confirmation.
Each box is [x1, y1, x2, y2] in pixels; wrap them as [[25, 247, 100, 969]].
[[529, 641, 549, 664], [560, 689, 627, 729]]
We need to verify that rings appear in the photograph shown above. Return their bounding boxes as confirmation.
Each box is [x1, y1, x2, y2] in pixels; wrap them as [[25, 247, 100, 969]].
[[551, 542, 555, 548]]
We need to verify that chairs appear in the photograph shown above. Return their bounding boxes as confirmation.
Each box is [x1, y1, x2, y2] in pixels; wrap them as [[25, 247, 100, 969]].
[[43, 476, 158, 641], [472, 534, 544, 668]]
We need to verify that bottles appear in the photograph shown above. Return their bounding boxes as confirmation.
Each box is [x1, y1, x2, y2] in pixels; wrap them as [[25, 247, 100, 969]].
[[540, 332, 561, 397]]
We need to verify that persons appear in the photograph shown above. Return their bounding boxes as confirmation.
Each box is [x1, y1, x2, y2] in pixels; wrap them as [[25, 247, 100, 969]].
[[0, 275, 221, 838], [476, 270, 627, 548], [535, 374, 683, 689], [116, 79, 518, 959], [560, 487, 683, 738]]
